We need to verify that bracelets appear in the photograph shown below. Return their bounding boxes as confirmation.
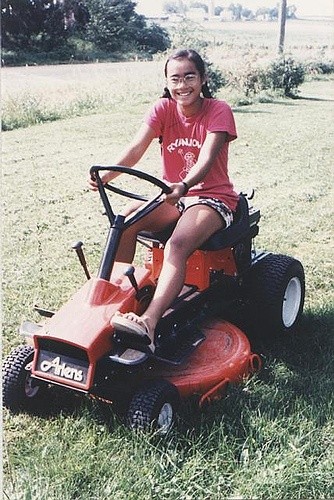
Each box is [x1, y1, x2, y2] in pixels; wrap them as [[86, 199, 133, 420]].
[[180, 181, 189, 196]]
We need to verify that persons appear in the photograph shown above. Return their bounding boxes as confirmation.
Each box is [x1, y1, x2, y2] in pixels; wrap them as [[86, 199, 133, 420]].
[[88, 49, 240, 354]]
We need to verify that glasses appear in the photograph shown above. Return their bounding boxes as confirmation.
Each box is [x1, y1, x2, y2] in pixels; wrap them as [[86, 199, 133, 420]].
[[168, 75, 201, 87]]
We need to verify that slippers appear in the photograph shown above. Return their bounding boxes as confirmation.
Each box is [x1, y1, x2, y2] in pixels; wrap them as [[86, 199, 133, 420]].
[[109, 311, 156, 354]]
